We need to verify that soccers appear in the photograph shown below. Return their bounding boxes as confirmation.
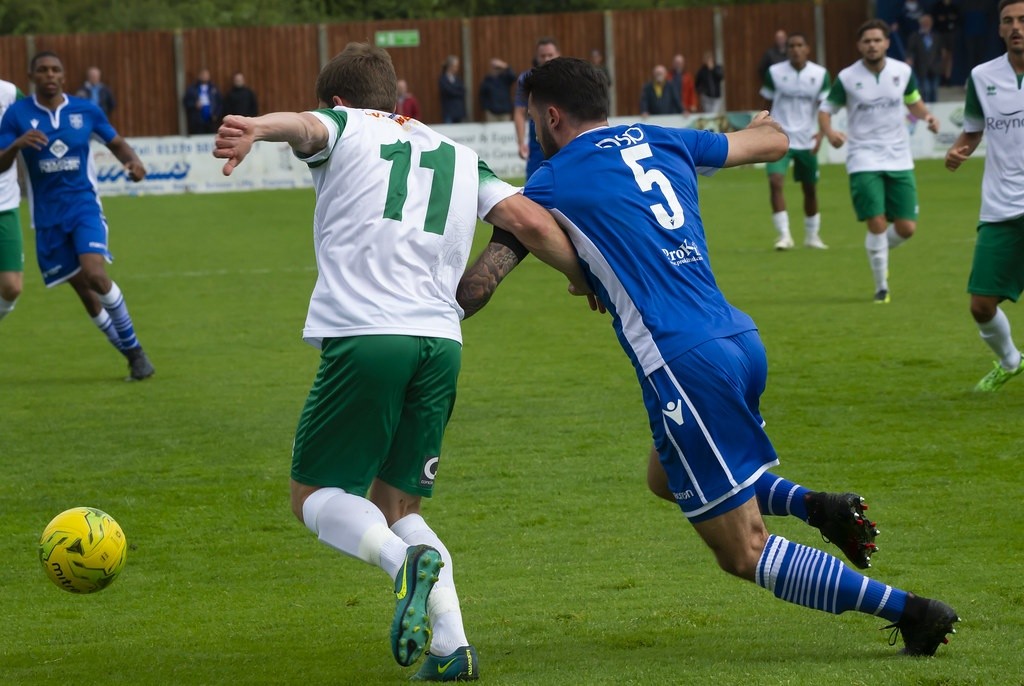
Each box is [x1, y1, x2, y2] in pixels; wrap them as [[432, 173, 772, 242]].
[[39, 506, 127, 594]]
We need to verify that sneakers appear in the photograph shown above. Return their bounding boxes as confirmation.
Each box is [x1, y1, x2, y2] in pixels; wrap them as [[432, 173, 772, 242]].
[[874, 290, 889, 304], [879, 591, 962, 656], [974, 351, 1024, 392], [773, 238, 794, 250], [804, 491, 880, 570], [390, 543, 445, 667], [804, 240, 828, 250], [130, 352, 154, 381], [409, 645, 480, 681]]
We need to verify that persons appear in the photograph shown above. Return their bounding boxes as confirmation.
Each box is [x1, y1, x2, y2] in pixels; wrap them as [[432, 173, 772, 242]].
[[210, 38, 609, 680], [761, 31, 831, 251], [1, 51, 156, 380], [887, 0, 951, 103], [455, 54, 962, 659], [943, 0, 1024, 395], [183, 68, 223, 134], [817, 20, 940, 306]]
